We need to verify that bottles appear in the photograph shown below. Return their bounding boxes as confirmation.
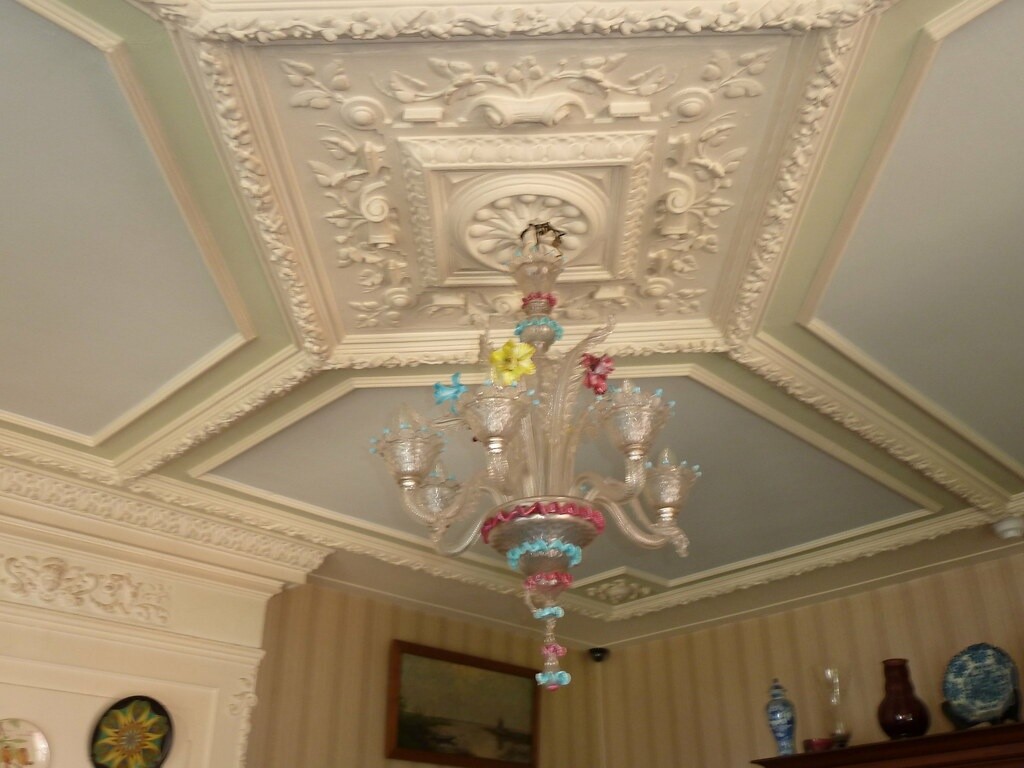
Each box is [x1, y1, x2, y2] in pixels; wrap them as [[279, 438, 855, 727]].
[[766, 677, 797, 755], [826, 667, 853, 748], [877, 659, 930, 741]]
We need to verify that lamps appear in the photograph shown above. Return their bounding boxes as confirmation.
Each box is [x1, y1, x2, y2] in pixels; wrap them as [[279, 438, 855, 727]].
[[371, 223, 704, 690]]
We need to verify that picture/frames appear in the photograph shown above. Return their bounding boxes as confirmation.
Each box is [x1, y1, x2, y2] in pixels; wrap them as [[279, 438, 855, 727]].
[[386, 638, 543, 768]]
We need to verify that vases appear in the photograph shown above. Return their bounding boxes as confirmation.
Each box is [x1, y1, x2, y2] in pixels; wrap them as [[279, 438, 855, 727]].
[[877, 657, 930, 741]]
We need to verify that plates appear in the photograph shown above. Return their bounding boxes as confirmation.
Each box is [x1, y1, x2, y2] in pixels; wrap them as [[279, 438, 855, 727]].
[[89, 695, 173, 768], [942, 642, 1019, 723], [0, 718, 51, 768]]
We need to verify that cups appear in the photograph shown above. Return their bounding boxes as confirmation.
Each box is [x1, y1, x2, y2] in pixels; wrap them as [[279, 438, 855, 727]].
[[803, 738, 832, 755]]
